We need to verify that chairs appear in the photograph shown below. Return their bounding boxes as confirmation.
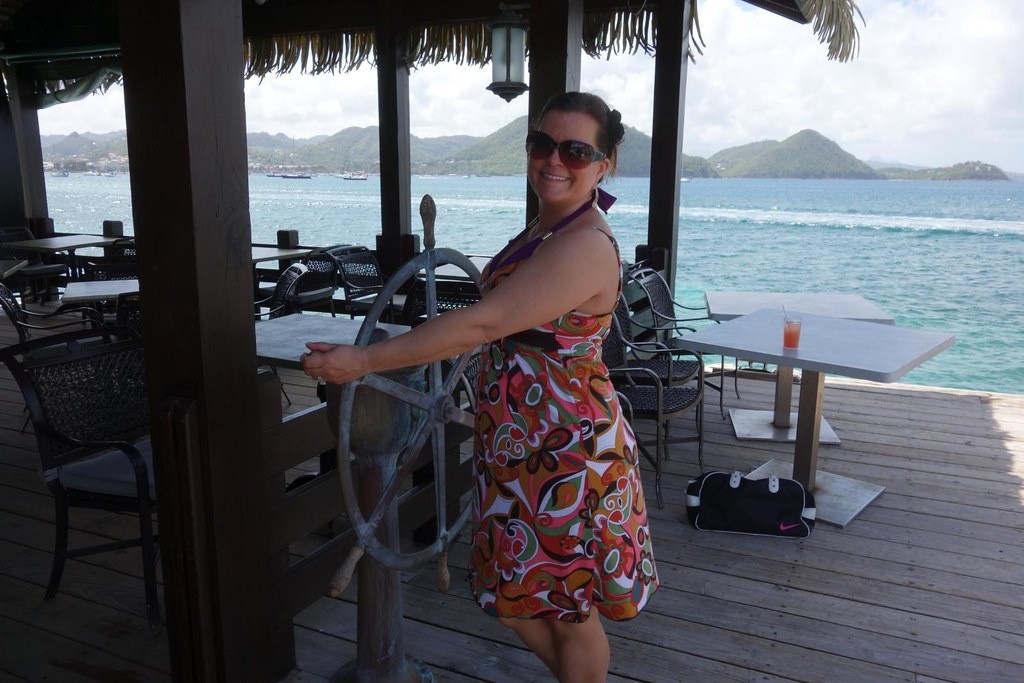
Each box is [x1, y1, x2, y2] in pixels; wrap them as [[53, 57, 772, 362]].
[[0, 225, 960, 633]]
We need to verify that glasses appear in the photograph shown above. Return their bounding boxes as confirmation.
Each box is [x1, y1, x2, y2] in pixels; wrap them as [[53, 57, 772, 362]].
[[525, 129, 608, 170]]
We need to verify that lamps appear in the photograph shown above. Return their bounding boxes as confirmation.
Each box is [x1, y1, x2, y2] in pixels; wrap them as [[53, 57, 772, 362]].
[[483, 2, 529, 102]]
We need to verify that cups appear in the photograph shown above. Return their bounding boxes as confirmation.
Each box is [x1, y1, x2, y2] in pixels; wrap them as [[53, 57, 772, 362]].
[[784, 314, 802, 349]]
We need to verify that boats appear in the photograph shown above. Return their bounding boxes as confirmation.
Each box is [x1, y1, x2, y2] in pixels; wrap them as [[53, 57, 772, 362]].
[[267, 170, 367, 180]]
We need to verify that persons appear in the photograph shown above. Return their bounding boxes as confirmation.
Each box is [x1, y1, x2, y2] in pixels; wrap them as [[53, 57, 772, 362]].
[[301, 93, 663, 683]]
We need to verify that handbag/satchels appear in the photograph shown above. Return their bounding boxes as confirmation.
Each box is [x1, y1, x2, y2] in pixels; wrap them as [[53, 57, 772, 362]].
[[684, 470, 817, 538]]
